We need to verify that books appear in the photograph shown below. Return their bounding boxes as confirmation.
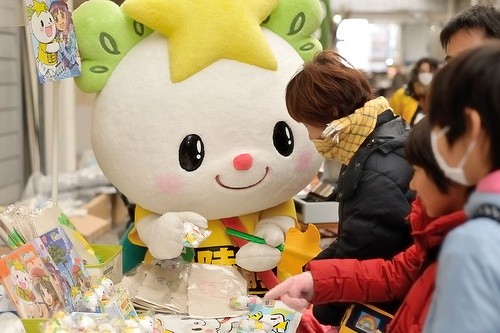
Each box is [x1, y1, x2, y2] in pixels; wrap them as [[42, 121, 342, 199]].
[[0, 201, 103, 318]]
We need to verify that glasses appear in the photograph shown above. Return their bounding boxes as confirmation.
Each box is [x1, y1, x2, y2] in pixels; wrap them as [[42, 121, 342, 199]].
[[412, 93, 427, 102]]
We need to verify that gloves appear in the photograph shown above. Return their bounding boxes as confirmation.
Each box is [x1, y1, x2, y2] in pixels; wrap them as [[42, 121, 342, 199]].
[[264, 271, 314, 312]]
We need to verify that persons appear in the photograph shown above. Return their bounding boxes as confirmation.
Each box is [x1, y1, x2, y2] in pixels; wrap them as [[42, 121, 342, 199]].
[[264, 5, 500, 332]]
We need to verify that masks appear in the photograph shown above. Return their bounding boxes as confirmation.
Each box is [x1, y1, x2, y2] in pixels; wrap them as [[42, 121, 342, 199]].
[[418, 73, 433, 85], [430, 126, 476, 186]]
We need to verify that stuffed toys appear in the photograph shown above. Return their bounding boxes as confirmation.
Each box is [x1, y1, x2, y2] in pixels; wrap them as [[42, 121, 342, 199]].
[[69, 0, 328, 299]]
